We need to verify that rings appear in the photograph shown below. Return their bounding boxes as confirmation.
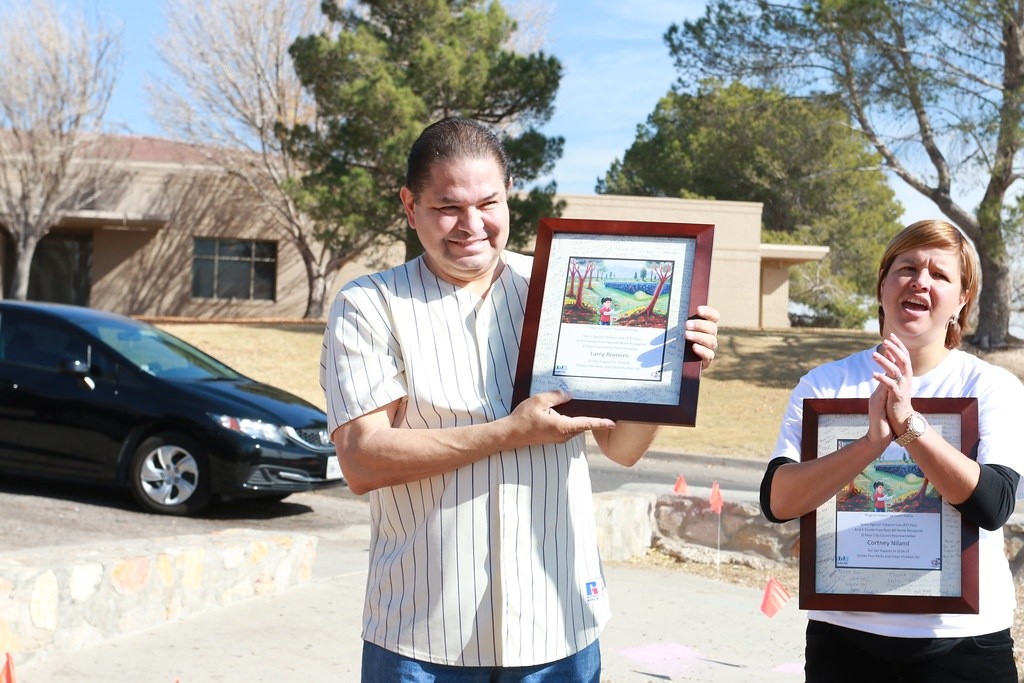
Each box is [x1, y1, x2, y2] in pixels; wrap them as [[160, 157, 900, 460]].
[[893, 374, 904, 384]]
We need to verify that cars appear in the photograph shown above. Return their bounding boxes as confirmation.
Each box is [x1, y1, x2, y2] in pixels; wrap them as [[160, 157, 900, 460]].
[[0, 294, 344, 514]]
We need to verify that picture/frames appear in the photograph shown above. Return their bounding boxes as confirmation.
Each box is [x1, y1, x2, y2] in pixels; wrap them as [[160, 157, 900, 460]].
[[797, 398, 981, 616], [508, 218, 716, 428]]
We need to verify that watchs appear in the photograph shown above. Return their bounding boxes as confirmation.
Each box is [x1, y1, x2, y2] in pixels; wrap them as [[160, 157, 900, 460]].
[[893, 411, 930, 448]]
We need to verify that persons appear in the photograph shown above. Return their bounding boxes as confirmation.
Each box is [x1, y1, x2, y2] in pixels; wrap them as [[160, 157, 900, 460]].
[[322, 116, 718, 683], [761, 219, 1024, 683]]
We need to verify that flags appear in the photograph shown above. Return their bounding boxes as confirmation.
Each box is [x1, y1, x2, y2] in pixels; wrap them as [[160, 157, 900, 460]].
[[674, 473, 725, 515], [762, 577, 792, 618]]
